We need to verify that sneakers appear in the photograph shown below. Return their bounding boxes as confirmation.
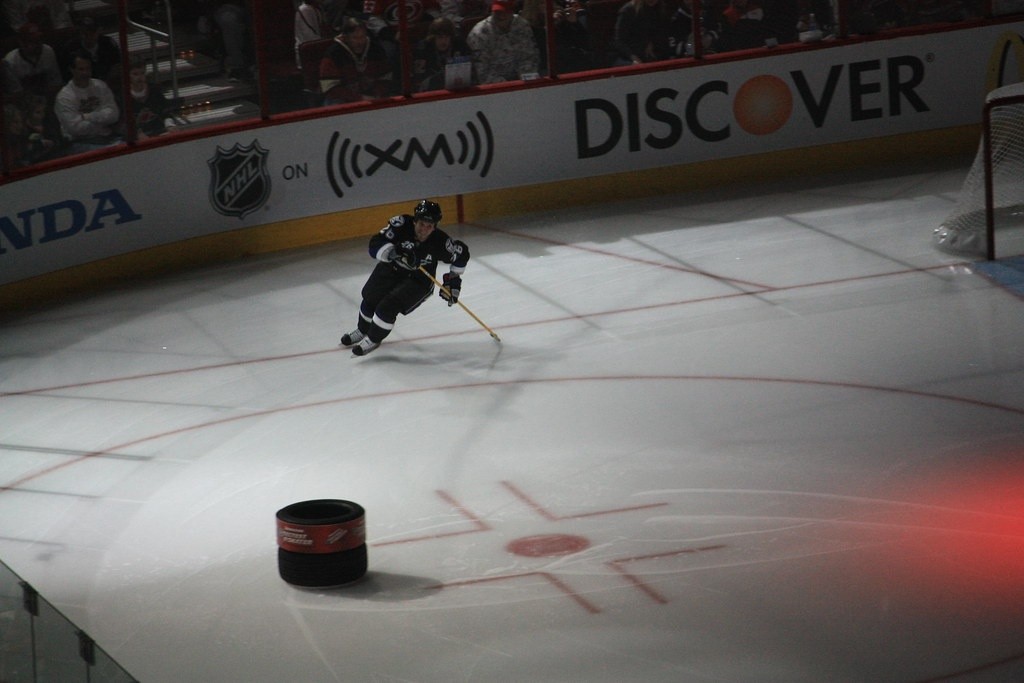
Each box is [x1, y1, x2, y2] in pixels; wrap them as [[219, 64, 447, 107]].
[[339, 329, 365, 346], [351, 336, 381, 359]]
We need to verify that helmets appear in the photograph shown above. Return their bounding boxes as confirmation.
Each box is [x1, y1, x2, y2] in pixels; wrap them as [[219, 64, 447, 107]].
[[414, 200, 442, 222]]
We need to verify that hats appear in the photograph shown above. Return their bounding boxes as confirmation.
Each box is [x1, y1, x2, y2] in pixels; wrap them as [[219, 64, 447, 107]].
[[21, 23, 44, 50], [492, 0, 516, 13]]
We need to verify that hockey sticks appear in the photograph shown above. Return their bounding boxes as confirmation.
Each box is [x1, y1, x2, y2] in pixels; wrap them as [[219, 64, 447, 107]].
[[418, 264, 503, 343]]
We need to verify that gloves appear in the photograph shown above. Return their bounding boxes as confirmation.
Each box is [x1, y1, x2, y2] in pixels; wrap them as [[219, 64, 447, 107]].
[[394, 242, 421, 271], [439, 271, 462, 307]]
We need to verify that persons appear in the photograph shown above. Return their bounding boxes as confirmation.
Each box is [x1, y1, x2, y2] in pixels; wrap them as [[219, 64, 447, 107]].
[[0, 0, 1024, 170], [341, 200, 470, 356]]
